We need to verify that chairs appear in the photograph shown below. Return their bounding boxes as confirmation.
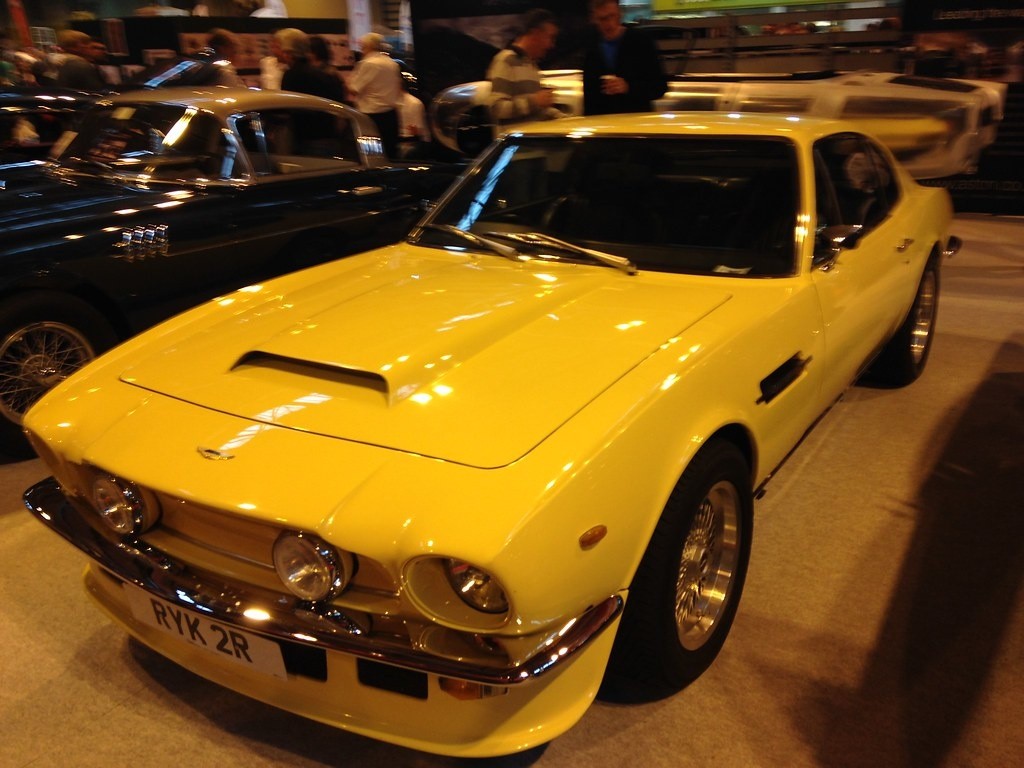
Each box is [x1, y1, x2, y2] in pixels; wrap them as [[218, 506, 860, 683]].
[[550, 191, 669, 243]]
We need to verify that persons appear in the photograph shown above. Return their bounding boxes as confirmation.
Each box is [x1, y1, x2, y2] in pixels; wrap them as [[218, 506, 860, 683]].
[[0, 0, 401, 162], [487, 0, 904, 142]]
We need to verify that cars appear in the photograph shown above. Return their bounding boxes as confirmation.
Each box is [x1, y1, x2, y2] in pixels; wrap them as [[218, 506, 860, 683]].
[[424, 50, 1013, 216], [1, 90, 462, 451], [19, 108, 956, 759]]
